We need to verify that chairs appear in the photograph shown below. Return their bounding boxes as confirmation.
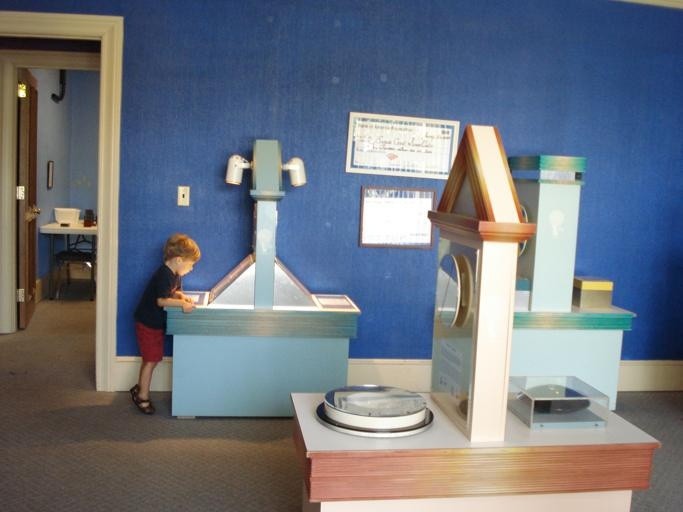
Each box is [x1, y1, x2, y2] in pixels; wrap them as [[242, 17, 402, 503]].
[[52, 235, 95, 301]]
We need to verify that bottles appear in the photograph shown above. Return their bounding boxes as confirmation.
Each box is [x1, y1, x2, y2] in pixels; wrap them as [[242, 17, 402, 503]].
[[83, 208, 95, 227]]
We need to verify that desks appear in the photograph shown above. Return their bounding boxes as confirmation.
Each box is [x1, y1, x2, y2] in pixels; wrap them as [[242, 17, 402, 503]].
[[39, 219, 98, 302]]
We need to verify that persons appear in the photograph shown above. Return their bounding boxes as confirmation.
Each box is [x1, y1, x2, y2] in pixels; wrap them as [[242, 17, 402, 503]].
[[128, 233, 201, 417]]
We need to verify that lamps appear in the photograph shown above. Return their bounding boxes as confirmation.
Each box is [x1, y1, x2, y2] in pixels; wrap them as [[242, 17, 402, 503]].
[[223, 154, 252, 186], [281, 156, 308, 189]]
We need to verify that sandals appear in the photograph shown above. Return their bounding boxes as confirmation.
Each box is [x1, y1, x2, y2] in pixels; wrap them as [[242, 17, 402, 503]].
[[132, 394, 155, 414], [131, 384, 138, 395]]
